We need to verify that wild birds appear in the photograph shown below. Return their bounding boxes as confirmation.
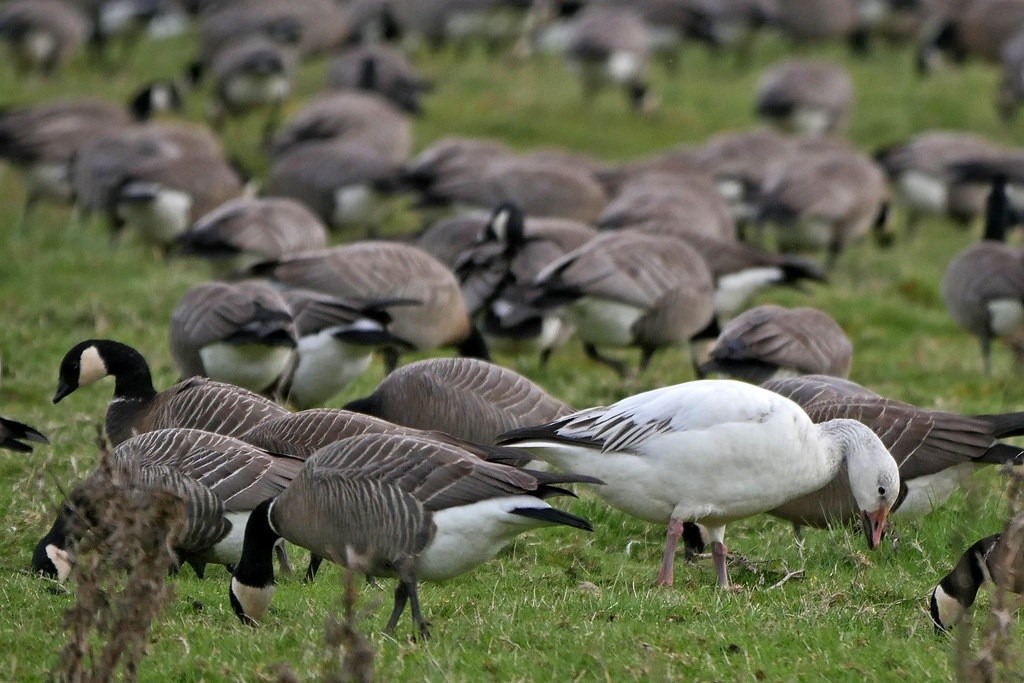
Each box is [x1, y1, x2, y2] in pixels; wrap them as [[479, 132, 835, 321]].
[[0, 1, 1024, 644]]
